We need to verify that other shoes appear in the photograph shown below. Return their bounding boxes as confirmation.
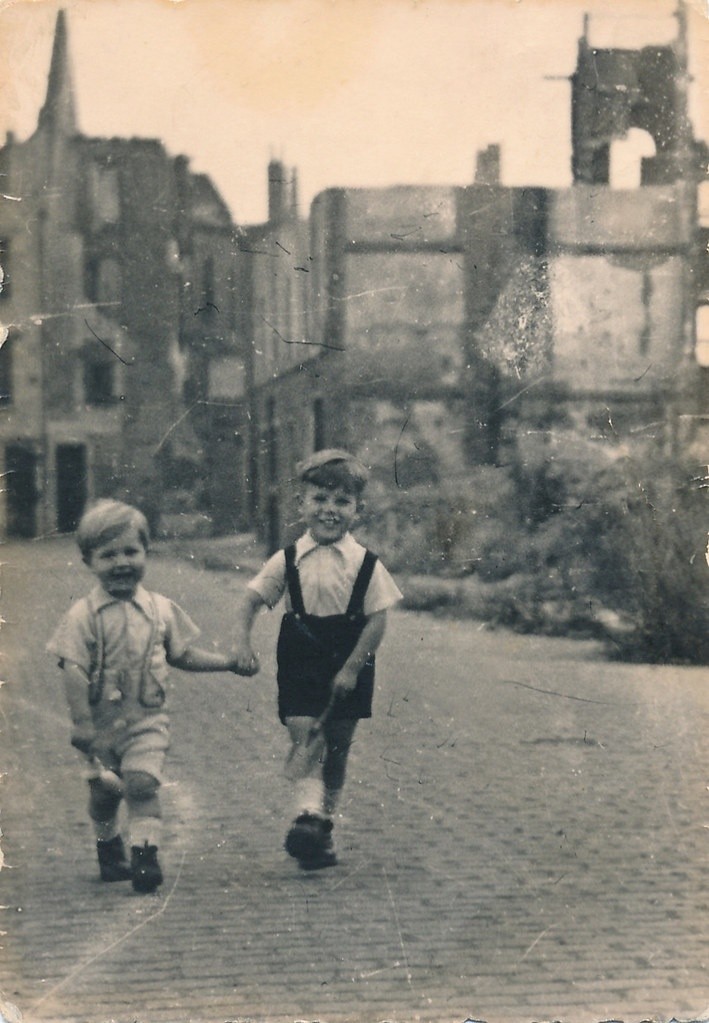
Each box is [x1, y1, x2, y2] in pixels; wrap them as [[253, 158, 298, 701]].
[[286, 813, 324, 859], [96, 831, 132, 882], [132, 839, 164, 894], [299, 834, 337, 870]]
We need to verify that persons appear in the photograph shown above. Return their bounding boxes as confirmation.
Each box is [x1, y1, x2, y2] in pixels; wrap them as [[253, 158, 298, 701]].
[[44, 501, 261, 893], [234, 449, 404, 870]]
